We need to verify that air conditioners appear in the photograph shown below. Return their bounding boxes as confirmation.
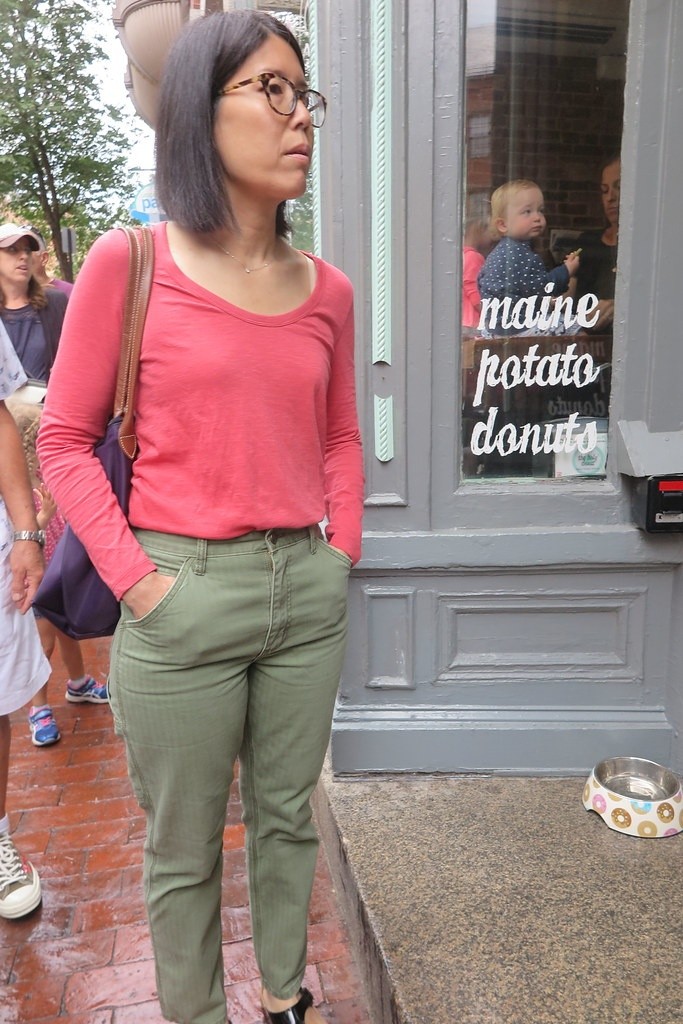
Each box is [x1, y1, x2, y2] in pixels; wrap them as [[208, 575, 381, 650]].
[[496, 10, 623, 57]]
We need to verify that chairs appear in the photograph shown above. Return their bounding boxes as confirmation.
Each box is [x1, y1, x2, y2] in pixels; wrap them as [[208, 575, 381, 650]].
[[463, 333, 612, 477]]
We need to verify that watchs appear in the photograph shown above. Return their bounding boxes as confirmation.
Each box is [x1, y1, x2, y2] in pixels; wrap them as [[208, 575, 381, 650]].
[[13, 529, 46, 546]]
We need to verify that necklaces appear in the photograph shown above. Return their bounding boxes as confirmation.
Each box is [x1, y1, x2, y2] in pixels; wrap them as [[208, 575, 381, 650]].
[[207, 232, 274, 273]]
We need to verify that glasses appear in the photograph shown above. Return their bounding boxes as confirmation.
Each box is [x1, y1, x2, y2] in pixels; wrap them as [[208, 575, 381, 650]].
[[213, 72, 327, 128]]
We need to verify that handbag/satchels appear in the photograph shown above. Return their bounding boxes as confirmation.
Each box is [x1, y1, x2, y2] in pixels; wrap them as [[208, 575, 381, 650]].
[[28, 227, 155, 640]]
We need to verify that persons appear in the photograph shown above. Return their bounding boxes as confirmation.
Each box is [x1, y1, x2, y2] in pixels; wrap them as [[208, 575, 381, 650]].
[[459, 154, 620, 478], [35, 8, 368, 1024], [0, 222, 111, 920]]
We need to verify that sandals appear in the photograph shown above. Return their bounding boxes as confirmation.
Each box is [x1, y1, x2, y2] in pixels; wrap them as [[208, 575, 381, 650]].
[[257, 982, 314, 1024]]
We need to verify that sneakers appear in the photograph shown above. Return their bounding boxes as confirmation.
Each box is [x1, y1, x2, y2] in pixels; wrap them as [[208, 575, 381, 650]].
[[65, 674, 109, 704], [0, 833, 42, 920], [29, 706, 60, 746]]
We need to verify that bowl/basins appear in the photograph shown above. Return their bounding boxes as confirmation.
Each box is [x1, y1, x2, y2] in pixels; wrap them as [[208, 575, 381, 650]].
[[582, 756, 683, 839]]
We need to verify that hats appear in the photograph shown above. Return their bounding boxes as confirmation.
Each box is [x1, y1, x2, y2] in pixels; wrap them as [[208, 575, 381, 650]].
[[0, 223, 39, 252]]
